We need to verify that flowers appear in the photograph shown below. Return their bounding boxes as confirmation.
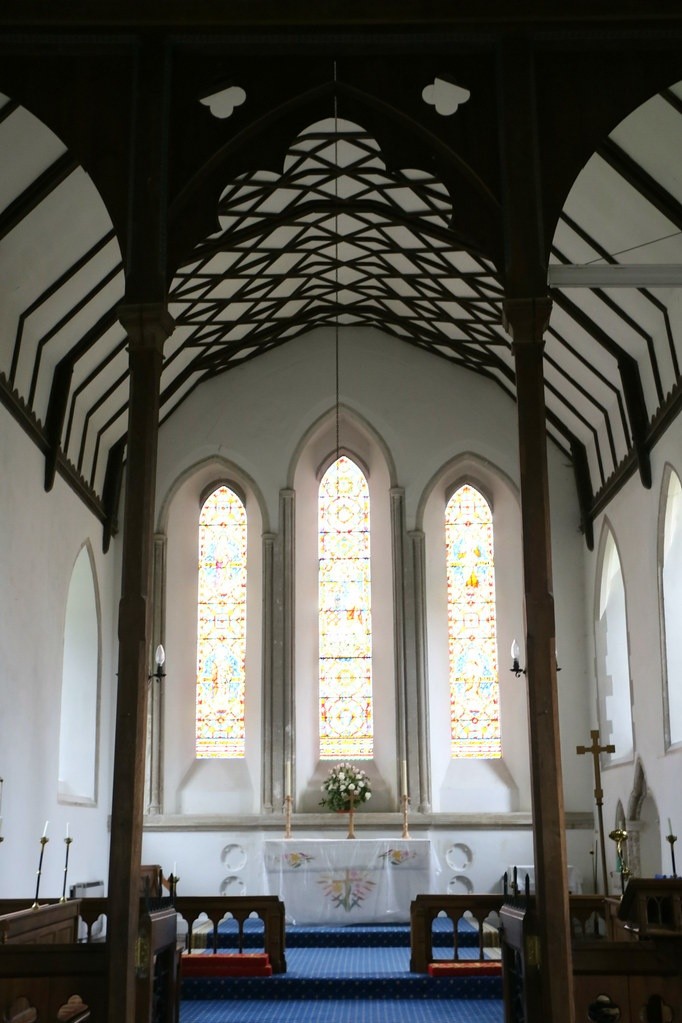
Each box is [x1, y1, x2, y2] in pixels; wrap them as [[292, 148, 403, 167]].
[[318, 762, 373, 812]]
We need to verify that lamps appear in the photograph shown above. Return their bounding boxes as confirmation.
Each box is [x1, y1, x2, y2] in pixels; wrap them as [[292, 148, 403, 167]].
[[422, 79, 470, 116], [509, 638, 527, 678], [547, 263, 682, 289], [200, 87, 247, 119], [146, 643, 168, 687]]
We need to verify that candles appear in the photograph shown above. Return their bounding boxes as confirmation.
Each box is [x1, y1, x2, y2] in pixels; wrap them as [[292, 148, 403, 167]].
[[285, 761, 292, 800], [42, 821, 50, 839], [65, 822, 71, 840], [616, 821, 621, 829], [667, 819, 675, 837], [401, 761, 409, 799]]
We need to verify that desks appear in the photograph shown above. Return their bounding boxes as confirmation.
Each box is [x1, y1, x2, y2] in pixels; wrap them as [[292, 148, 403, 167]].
[[510, 863, 583, 895], [262, 836, 436, 926]]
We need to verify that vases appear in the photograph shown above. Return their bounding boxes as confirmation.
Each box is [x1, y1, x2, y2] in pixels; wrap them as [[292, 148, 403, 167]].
[[334, 806, 351, 815]]
